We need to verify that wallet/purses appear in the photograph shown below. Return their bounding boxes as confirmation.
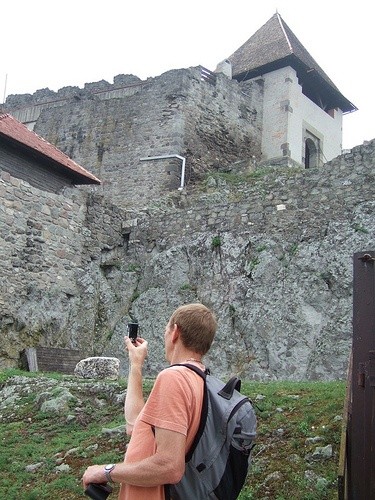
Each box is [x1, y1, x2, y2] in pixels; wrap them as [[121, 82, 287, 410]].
[[81, 478, 112, 499]]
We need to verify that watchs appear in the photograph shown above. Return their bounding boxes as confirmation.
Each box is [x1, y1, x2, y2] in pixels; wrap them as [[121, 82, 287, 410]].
[[104, 464, 115, 484]]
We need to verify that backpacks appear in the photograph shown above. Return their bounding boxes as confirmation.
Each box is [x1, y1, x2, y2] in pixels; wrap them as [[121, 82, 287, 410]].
[[158, 360, 258, 500]]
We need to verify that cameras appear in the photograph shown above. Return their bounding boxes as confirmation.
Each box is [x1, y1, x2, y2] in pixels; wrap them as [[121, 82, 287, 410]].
[[127, 323, 139, 343]]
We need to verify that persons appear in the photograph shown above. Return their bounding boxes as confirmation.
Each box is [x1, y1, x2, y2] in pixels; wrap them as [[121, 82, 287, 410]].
[[83, 303, 216, 500]]
